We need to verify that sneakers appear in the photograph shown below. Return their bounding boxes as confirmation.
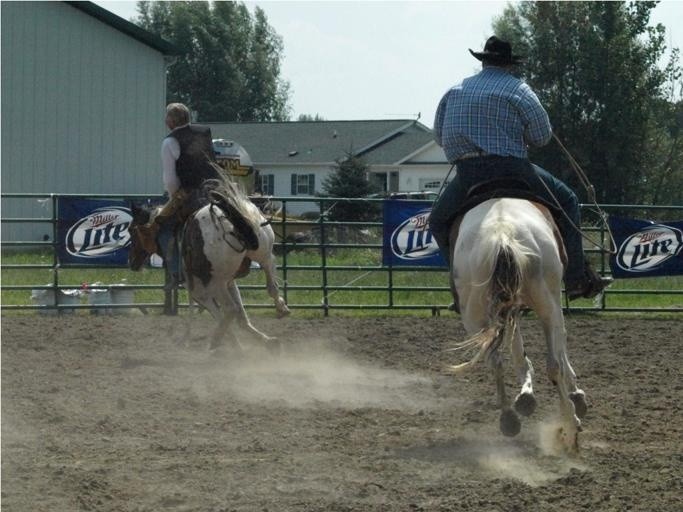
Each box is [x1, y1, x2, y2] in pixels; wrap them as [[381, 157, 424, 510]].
[[448, 302, 455, 311], [563, 278, 613, 295]]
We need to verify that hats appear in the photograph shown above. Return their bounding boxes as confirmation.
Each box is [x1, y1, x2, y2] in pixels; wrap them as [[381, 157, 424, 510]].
[[468, 36, 528, 64]]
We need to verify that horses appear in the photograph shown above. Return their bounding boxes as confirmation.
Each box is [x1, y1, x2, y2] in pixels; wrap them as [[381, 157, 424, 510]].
[[450, 197, 587, 436], [128, 153, 291, 360]]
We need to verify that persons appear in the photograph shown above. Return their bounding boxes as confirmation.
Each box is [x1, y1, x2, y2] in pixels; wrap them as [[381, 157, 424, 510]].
[[158, 97, 225, 293], [425, 34, 616, 312]]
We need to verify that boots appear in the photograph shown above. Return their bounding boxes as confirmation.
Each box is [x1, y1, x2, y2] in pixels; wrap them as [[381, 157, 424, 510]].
[[154, 201, 196, 232]]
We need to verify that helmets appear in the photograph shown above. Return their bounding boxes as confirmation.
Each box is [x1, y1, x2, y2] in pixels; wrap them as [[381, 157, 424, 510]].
[[163, 103, 191, 131]]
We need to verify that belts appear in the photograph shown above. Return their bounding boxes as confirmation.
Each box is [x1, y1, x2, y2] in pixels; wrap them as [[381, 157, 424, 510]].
[[457, 150, 494, 159]]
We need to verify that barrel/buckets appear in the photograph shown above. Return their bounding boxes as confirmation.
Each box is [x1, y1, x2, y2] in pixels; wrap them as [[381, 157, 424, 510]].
[[56, 285, 135, 316]]
